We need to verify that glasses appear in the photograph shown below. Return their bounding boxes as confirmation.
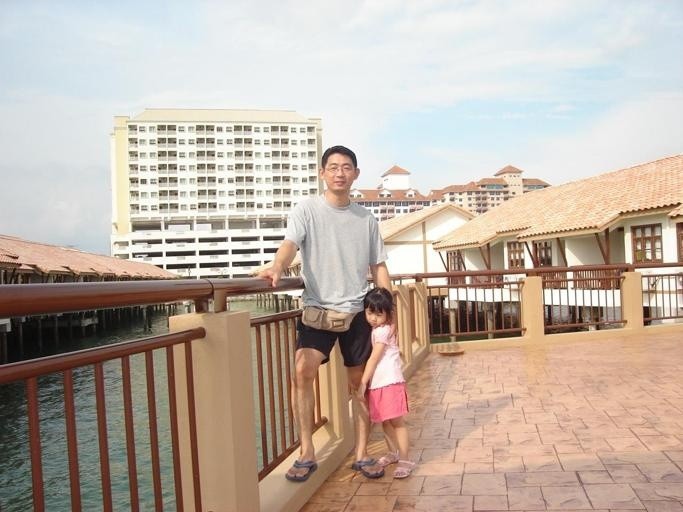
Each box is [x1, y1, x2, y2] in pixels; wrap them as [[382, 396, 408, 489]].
[[325, 165, 357, 174]]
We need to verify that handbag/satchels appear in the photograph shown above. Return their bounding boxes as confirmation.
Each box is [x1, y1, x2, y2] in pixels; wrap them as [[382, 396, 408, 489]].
[[301, 304, 354, 332]]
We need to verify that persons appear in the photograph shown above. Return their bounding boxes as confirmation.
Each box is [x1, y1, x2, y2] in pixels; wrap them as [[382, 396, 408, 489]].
[[255, 146, 397, 481], [356, 288, 417, 479]]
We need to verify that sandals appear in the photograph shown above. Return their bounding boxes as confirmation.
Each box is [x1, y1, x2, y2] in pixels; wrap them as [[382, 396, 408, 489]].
[[377, 449, 415, 479]]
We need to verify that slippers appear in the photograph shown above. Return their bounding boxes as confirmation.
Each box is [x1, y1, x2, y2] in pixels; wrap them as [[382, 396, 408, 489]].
[[286, 459, 317, 483], [349, 456, 383, 479]]
